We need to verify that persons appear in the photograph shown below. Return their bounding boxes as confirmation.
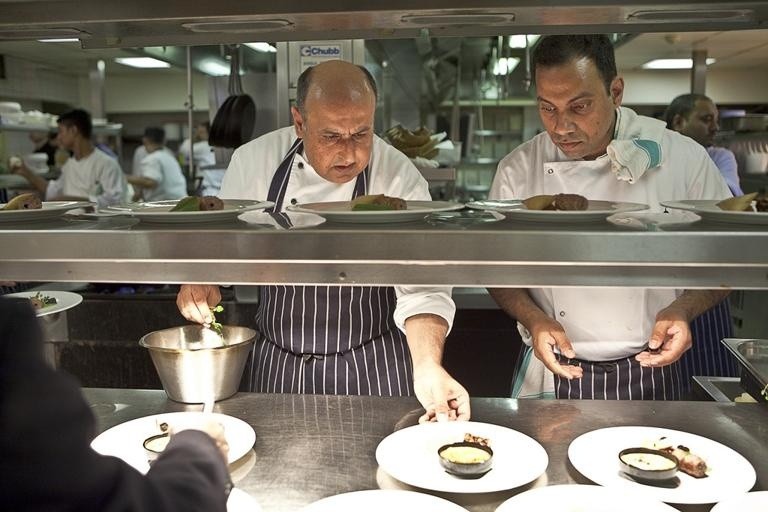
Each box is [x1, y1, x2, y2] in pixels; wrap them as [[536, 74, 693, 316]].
[[665, 91, 745, 199], [175, 58, 473, 424], [479, 33, 745, 400], [7, 108, 223, 212], [0, 293, 235, 512]]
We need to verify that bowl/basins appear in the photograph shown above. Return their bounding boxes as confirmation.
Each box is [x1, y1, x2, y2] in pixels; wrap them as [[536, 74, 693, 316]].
[[437, 440, 495, 476], [614, 445, 681, 483], [137, 324, 261, 404]]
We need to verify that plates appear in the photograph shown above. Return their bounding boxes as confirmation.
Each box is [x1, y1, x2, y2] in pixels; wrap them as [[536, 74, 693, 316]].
[[465, 200, 650, 223], [72, 207, 130, 218], [88, 411, 256, 476], [493, 486, 675, 511], [304, 491, 469, 511], [0, 289, 83, 318], [142, 431, 171, 457], [1, 200, 94, 223], [606, 209, 703, 225], [237, 211, 327, 226], [566, 427, 756, 503], [119, 200, 274, 222], [374, 421, 548, 494], [660, 198, 768, 224], [426, 210, 507, 223], [710, 491, 767, 510], [287, 201, 466, 221]]
